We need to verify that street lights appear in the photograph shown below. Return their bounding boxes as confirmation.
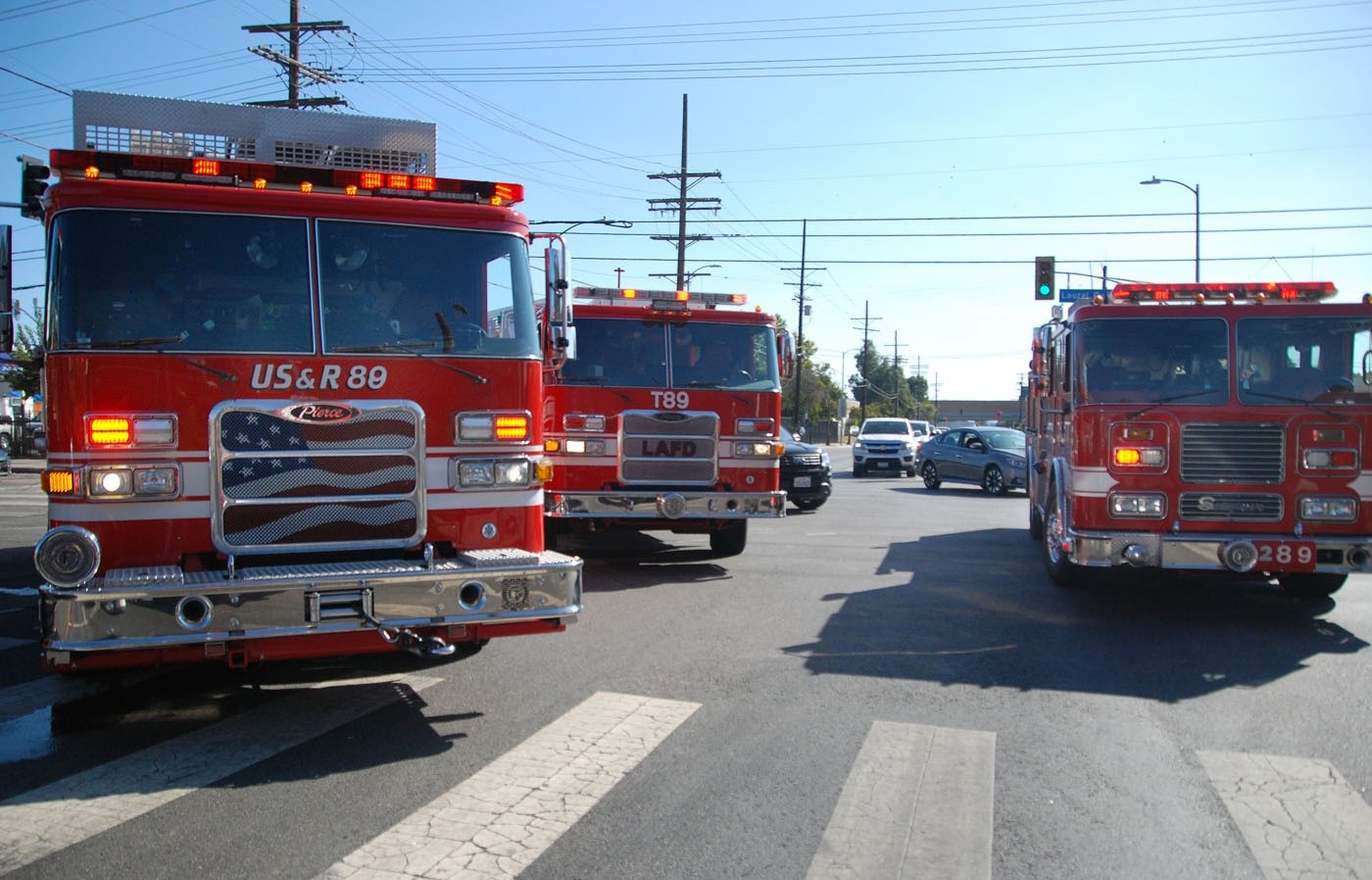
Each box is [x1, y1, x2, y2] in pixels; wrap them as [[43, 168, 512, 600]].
[[1139, 175, 1200, 282]]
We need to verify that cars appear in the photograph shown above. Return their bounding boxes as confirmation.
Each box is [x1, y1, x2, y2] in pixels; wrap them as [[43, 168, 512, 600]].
[[914, 426, 1028, 497], [909, 419, 952, 449]]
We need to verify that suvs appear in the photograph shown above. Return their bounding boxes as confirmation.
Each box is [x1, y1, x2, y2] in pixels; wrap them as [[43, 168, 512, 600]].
[[848, 417, 922, 477], [777, 422, 834, 510]]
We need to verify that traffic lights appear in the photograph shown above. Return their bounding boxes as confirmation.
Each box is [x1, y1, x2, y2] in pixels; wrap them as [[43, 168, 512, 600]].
[[1035, 256, 1055, 300]]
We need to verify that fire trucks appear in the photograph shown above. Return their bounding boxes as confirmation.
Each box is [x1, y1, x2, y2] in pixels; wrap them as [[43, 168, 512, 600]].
[[1, 88, 584, 682], [485, 285, 797, 561], [1025, 282, 1372, 598]]
